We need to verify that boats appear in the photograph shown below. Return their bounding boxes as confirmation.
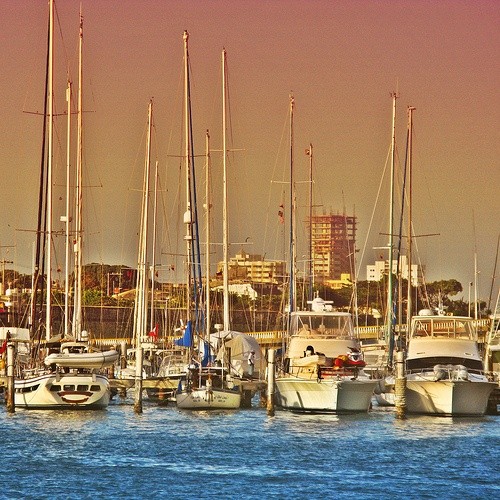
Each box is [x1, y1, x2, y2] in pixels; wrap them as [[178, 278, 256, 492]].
[[0, 288, 500, 416]]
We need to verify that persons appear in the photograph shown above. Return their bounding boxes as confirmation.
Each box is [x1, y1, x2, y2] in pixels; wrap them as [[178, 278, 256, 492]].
[[247, 349, 256, 379]]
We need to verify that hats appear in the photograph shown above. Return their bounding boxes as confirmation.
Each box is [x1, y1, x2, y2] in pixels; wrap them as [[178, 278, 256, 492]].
[[250, 349, 255, 352]]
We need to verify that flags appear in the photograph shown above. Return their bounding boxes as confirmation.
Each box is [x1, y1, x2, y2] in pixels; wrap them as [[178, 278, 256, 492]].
[[174, 321, 192, 346], [148, 322, 159, 344]]
